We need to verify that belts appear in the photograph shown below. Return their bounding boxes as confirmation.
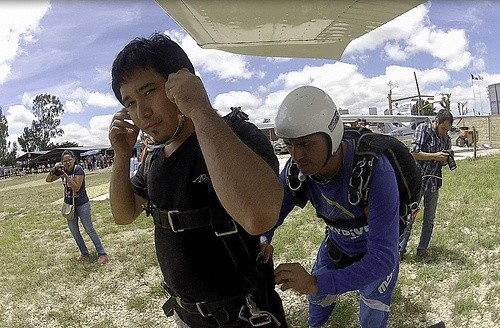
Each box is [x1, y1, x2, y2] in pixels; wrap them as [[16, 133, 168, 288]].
[[171, 285, 275, 316]]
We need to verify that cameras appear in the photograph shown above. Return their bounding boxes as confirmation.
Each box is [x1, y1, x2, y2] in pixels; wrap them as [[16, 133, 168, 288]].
[[55, 165, 65, 176], [442, 150, 457, 171]]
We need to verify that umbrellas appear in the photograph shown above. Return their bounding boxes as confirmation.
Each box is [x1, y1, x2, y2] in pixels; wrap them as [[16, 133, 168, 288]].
[[80, 150, 97, 156]]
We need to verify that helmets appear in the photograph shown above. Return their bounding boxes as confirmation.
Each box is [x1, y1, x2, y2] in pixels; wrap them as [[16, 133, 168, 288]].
[[274, 85, 344, 155]]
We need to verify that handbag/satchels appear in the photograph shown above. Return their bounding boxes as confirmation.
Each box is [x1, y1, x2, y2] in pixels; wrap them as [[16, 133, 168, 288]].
[[61, 203, 75, 220]]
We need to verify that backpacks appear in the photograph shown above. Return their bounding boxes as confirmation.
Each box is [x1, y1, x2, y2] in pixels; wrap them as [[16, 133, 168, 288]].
[[285, 126, 423, 222]]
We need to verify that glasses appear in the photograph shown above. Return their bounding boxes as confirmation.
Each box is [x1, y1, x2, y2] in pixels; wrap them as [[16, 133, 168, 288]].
[[63, 157, 74, 164]]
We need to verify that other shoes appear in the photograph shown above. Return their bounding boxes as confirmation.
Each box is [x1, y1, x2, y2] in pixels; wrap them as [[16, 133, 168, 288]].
[[415, 250, 428, 258], [398, 251, 404, 260], [97, 254, 107, 264], [77, 254, 89, 261]]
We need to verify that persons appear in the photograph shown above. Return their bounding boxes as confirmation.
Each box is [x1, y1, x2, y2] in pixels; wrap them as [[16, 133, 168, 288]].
[[261, 85, 399, 328], [46, 150, 107, 264], [15, 154, 104, 177], [109, 33, 288, 328], [399, 109, 454, 262]]
